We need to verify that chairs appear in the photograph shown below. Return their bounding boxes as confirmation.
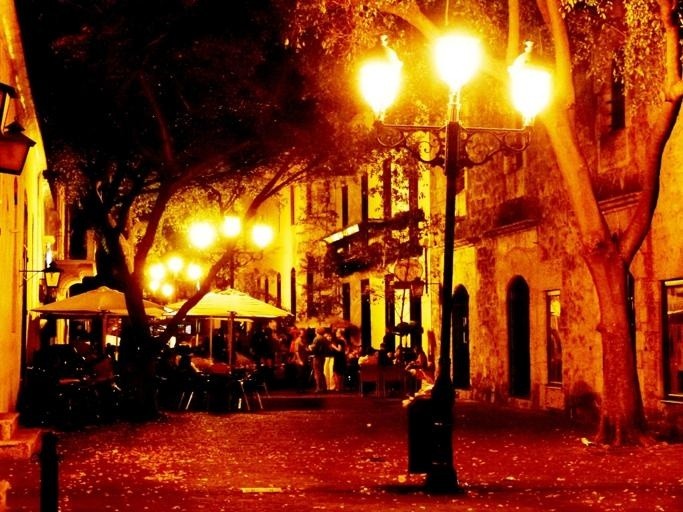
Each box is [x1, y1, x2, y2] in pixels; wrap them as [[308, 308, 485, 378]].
[[46, 344, 423, 413]]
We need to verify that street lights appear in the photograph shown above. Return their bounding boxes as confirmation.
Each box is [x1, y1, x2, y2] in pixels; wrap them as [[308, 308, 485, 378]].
[[356, 25, 560, 494], [148, 215, 272, 368]]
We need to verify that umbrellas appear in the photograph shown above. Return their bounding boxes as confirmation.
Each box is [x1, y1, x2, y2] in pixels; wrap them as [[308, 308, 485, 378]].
[[164, 286, 295, 364], [29, 285, 171, 349]]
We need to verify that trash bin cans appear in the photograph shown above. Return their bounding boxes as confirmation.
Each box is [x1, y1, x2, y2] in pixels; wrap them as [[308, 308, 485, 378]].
[[407, 396, 433, 474]]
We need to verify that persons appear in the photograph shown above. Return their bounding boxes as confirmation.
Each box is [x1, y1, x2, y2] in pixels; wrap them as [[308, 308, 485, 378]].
[[190, 321, 428, 398]]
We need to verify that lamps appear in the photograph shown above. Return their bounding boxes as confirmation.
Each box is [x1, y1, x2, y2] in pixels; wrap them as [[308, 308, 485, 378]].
[[1, 120, 36, 177], [410, 276, 424, 297]]
